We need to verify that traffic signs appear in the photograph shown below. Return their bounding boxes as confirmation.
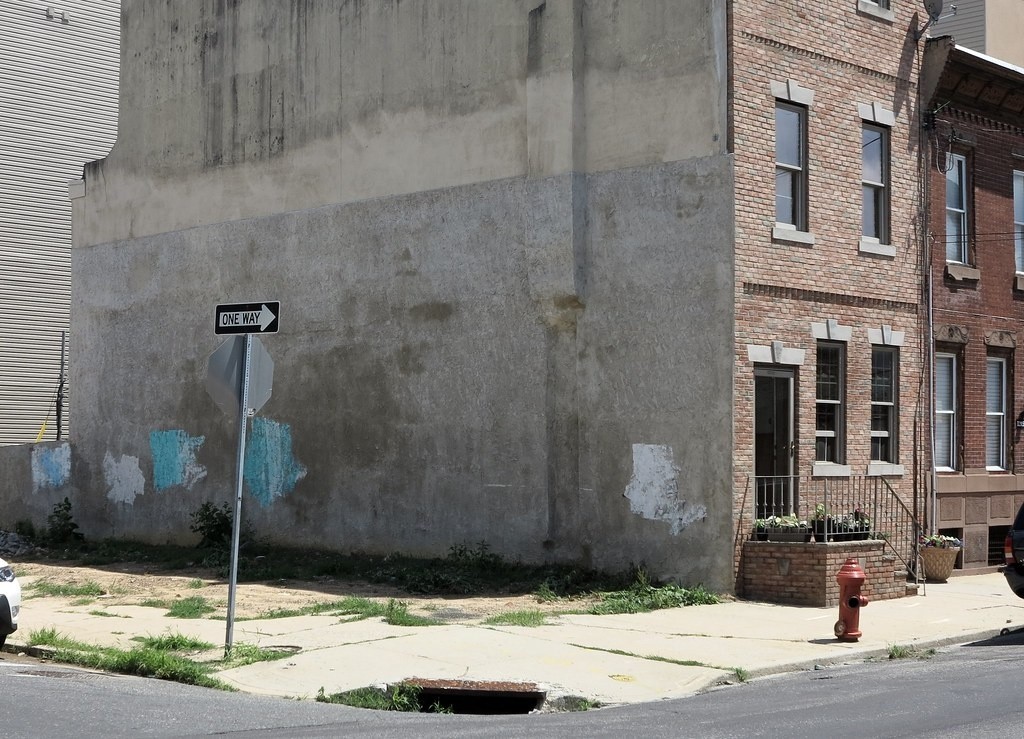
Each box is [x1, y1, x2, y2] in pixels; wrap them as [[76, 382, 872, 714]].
[[213, 303, 281, 335]]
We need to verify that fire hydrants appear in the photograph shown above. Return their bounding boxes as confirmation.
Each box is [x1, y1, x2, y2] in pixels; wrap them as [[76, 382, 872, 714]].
[[832, 558, 869, 643]]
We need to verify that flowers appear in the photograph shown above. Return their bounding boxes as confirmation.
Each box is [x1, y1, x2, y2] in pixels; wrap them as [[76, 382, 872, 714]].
[[919, 530, 962, 549], [850, 508, 870, 527]]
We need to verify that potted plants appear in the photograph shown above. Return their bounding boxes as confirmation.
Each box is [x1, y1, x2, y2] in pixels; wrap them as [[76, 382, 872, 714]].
[[765, 514, 812, 542], [752, 518, 768, 542], [811, 504, 833, 543], [832, 514, 854, 542]]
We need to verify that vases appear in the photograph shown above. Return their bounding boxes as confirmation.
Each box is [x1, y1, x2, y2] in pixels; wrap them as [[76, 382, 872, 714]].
[[919, 547, 960, 582], [854, 526, 863, 540]]
